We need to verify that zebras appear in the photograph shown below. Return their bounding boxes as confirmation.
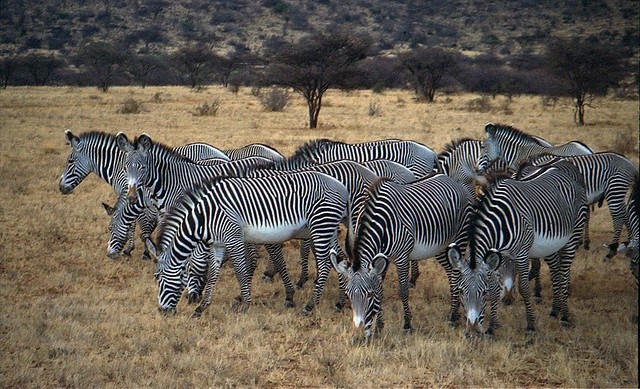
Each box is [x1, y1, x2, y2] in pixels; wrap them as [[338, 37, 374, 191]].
[[55, 119, 640, 348]]
